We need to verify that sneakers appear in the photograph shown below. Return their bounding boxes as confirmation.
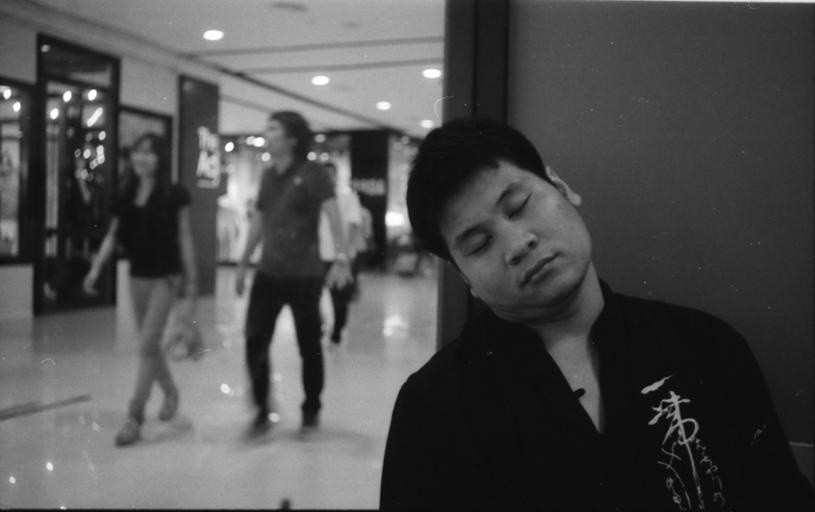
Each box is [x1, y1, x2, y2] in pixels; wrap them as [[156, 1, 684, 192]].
[[330, 326, 344, 346], [245, 404, 272, 439], [301, 412, 320, 434]]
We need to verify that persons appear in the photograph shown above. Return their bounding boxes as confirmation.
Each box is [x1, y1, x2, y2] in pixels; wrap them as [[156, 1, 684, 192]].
[[380, 116, 814, 511], [83, 131, 199, 445], [233, 111, 354, 441], [313, 160, 372, 344]]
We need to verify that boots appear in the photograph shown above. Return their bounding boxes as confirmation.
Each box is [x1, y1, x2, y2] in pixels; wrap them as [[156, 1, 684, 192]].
[[114, 399, 146, 446], [155, 371, 182, 422]]
